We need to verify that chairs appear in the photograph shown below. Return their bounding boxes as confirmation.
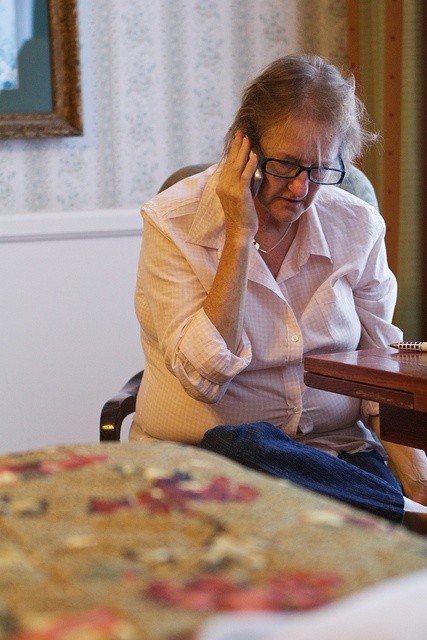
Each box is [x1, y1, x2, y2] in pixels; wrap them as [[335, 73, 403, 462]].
[[98, 160, 381, 442]]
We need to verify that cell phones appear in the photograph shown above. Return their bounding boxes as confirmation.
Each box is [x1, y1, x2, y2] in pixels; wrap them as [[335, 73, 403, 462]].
[[247, 144, 266, 203]]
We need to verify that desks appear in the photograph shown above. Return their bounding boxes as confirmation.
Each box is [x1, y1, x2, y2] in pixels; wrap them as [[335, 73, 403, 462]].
[[304, 342, 427, 412]]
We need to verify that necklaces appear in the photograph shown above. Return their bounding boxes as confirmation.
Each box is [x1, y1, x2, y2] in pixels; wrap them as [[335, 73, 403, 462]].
[[251, 221, 294, 254]]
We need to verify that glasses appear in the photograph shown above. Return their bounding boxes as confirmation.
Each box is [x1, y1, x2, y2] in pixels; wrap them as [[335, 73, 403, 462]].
[[250, 123, 346, 185]]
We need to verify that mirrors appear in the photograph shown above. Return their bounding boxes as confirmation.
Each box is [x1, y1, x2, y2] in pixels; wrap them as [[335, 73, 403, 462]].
[[1, 0, 88, 141]]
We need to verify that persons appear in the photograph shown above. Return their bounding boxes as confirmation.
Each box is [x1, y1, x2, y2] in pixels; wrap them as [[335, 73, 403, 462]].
[[127, 52, 427, 536]]
